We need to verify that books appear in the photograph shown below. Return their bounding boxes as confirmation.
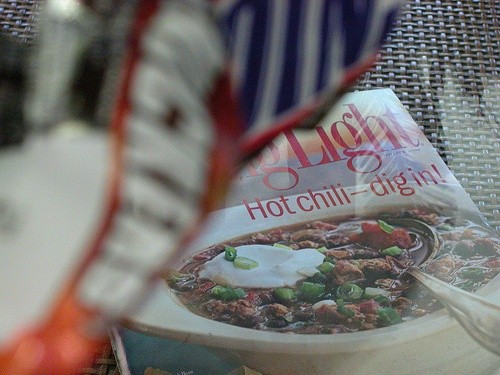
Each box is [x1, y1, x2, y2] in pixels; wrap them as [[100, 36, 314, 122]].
[[106, 86, 499, 375]]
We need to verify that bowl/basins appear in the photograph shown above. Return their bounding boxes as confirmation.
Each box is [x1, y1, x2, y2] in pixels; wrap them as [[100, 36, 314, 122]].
[[108, 179, 500, 354]]
